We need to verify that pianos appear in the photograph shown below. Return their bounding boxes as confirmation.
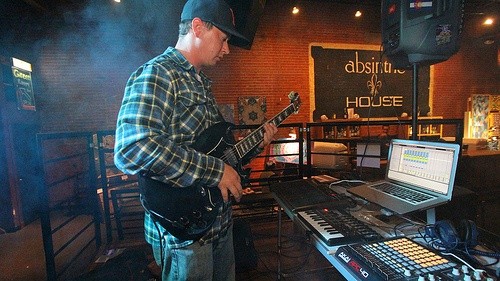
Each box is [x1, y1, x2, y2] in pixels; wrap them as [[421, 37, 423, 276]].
[[297, 205, 382, 251]]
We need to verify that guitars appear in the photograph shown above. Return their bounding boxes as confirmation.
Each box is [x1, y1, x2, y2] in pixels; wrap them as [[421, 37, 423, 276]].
[[140, 90, 305, 241]]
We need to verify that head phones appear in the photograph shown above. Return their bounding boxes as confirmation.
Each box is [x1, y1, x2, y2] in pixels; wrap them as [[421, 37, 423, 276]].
[[425, 219, 478, 250]]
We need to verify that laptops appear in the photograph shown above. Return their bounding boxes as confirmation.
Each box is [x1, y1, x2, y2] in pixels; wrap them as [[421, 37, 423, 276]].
[[347, 137, 461, 216]]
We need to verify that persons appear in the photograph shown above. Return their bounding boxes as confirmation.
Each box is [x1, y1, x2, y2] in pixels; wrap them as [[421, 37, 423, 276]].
[[114, 0, 279, 281]]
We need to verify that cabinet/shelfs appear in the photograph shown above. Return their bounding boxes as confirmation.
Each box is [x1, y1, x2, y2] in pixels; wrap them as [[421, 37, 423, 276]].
[[317, 117, 443, 165]]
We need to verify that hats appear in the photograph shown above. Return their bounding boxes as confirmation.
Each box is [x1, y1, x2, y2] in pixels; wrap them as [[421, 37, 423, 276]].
[[181, 0, 250, 48]]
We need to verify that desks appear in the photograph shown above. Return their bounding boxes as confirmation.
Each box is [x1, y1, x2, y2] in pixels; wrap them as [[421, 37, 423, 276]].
[[271, 186, 500, 281], [461, 143, 500, 226]]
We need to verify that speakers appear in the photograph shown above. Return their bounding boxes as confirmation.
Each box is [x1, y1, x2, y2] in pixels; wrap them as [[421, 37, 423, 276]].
[[381, 0, 464, 70], [224, 0, 266, 49]]
